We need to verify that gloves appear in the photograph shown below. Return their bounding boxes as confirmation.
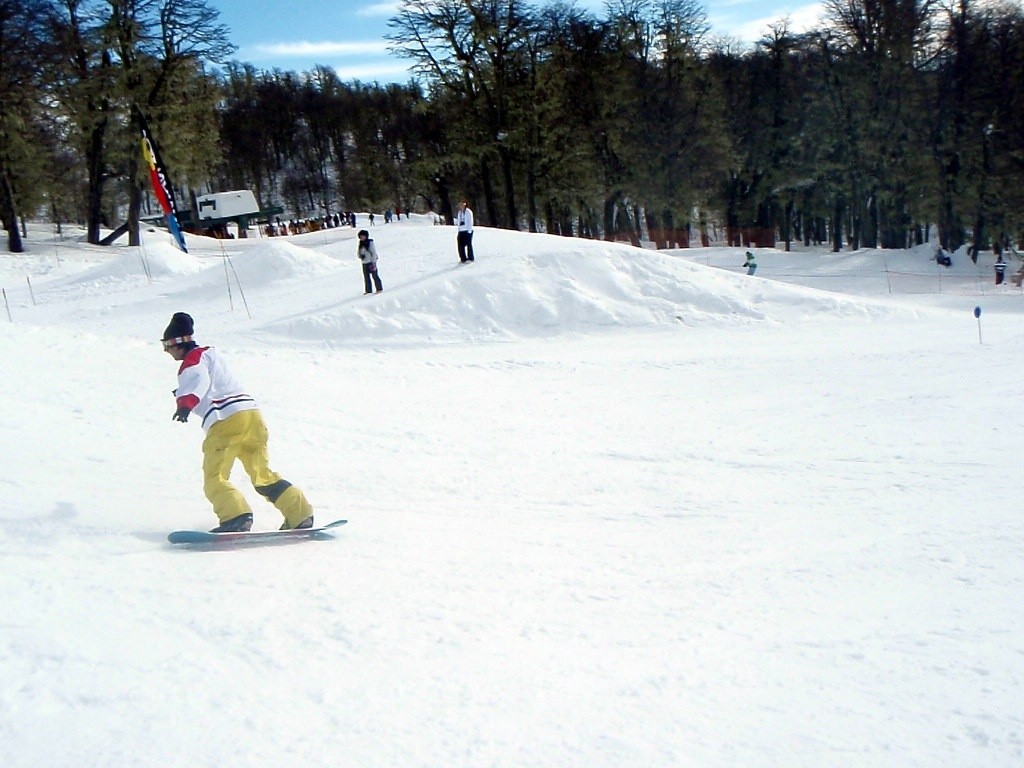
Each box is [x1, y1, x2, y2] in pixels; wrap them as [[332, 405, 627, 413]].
[[172, 389, 176, 396], [173, 406, 190, 423]]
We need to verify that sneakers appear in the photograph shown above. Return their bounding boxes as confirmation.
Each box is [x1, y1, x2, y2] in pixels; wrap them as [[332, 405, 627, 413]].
[[209, 513, 313, 533]]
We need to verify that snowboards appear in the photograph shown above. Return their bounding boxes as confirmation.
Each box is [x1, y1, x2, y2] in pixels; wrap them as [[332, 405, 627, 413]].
[[167, 520, 350, 542]]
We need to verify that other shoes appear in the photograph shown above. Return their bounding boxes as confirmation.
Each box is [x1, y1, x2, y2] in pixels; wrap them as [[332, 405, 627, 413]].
[[364, 291, 383, 296], [458, 260, 474, 266]]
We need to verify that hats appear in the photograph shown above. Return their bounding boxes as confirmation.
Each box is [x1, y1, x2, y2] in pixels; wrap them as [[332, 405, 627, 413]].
[[161, 312, 195, 346], [358, 230, 369, 240]]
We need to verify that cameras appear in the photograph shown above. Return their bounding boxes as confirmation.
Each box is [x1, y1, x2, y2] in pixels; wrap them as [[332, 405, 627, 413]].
[[743, 263, 749, 267], [460, 222, 465, 225]]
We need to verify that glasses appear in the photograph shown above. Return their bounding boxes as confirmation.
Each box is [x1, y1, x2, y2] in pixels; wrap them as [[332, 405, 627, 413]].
[[164, 344, 174, 351]]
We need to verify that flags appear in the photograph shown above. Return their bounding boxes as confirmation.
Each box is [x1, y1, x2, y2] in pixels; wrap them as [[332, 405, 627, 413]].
[[137, 118, 188, 255]]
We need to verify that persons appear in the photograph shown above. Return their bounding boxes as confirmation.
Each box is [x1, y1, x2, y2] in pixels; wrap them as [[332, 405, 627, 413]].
[[266, 209, 356, 237], [396, 205, 401, 220], [743, 252, 757, 275], [162, 313, 313, 533], [457, 200, 474, 262], [358, 230, 383, 293], [368, 212, 375, 225], [384, 209, 392, 222], [405, 205, 410, 217], [935, 239, 1007, 284]]
